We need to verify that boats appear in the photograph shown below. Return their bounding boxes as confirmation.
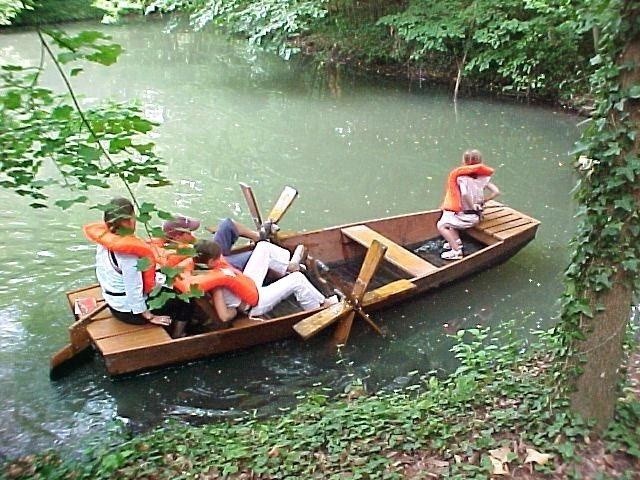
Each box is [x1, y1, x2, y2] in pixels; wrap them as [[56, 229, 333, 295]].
[[48, 181, 542, 382]]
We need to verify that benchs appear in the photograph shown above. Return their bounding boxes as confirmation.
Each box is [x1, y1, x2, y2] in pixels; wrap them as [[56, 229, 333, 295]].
[[341, 224, 436, 278], [188, 286, 262, 331]]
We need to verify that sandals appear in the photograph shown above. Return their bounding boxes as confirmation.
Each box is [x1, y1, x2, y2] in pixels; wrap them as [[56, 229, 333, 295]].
[[443, 239, 463, 249], [440, 250, 464, 260]]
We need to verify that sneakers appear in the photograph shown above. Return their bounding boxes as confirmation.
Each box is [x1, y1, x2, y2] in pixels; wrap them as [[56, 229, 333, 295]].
[[258, 221, 272, 241], [291, 245, 304, 272], [325, 295, 338, 304]]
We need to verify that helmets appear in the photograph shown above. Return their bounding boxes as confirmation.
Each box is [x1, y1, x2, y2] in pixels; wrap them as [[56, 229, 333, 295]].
[[463, 150, 482, 165]]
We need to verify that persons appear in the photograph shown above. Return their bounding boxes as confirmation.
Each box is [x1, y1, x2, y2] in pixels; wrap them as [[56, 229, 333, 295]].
[[189, 239, 342, 323], [436, 150, 499, 260], [155, 214, 274, 273], [96, 196, 193, 339]]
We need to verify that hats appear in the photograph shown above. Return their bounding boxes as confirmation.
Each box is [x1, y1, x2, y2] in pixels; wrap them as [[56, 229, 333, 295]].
[[162, 214, 200, 233]]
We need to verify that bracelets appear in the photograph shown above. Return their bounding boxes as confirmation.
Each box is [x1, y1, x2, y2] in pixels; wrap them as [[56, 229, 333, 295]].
[[147, 314, 154, 321]]
